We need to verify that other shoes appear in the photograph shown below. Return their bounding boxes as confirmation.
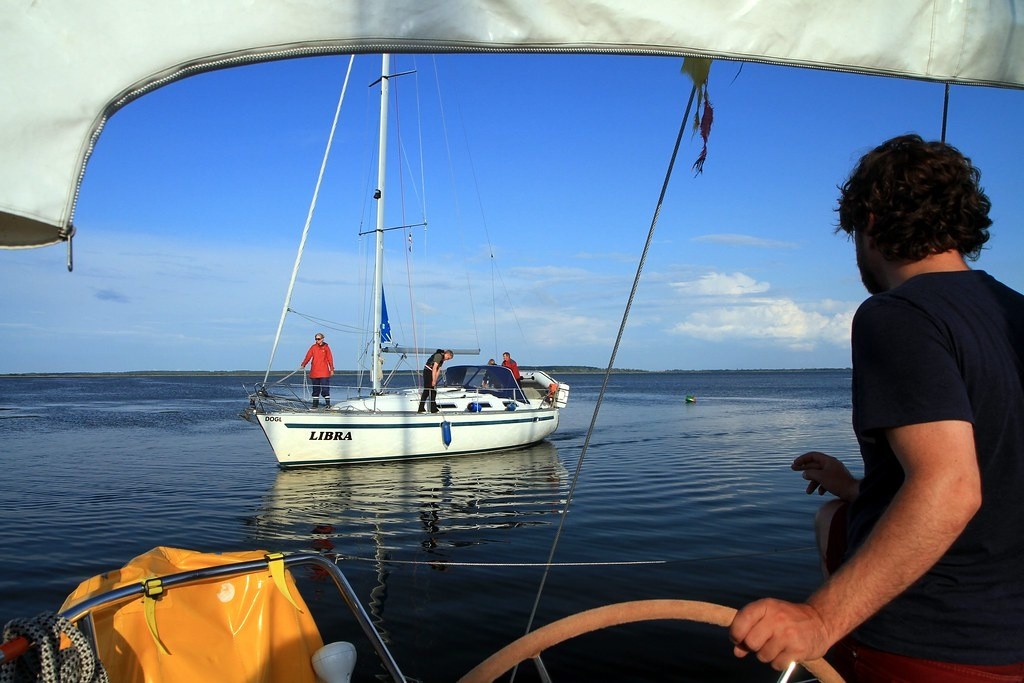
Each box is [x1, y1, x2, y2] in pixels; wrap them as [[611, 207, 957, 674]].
[[325, 404, 330, 409], [418, 409, 426, 413], [431, 409, 438, 413], [310, 405, 318, 409]]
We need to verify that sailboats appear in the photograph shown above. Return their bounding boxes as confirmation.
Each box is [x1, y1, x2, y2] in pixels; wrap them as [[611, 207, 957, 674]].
[[240, 53, 573, 468]]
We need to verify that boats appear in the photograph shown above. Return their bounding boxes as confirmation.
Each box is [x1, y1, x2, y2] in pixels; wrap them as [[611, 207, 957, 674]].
[[685, 395, 698, 402]]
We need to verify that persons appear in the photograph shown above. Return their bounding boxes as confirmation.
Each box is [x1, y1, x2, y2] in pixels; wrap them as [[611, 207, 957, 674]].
[[482, 352, 521, 400], [418, 349, 453, 414], [301, 334, 335, 410], [729, 134, 1024, 683]]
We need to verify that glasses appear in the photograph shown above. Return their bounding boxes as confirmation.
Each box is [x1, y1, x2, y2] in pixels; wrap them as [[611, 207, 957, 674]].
[[316, 338, 322, 341]]
